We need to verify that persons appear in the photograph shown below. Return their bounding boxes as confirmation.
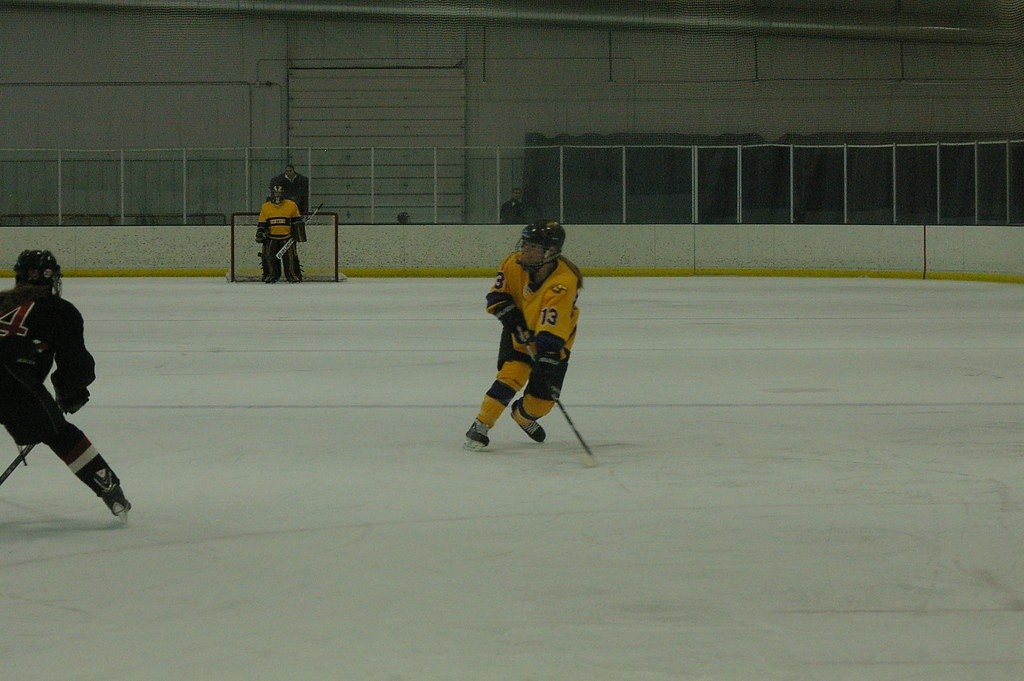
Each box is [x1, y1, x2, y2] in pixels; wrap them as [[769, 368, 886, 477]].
[[269, 165, 309, 215], [258, 185, 308, 283], [1, 249, 132, 526], [462, 220, 583, 450], [500, 189, 526, 224]]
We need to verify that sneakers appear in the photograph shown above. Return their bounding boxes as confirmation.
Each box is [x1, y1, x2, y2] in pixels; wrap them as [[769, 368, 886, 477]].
[[511, 397, 546, 442], [463, 418, 490, 451], [93, 468, 131, 525]]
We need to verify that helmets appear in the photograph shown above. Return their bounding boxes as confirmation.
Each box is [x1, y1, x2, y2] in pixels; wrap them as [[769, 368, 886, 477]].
[[13, 248, 63, 296], [270, 184, 284, 203], [515, 219, 565, 269]]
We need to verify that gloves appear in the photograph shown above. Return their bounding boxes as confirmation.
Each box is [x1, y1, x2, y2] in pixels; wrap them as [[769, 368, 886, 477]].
[[533, 351, 559, 393], [55, 392, 89, 414], [494, 303, 528, 336]]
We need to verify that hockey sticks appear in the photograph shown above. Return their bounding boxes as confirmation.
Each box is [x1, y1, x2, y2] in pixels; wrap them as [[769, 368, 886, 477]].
[[0, 443, 38, 486], [276, 203, 324, 260], [517, 327, 598, 471]]
